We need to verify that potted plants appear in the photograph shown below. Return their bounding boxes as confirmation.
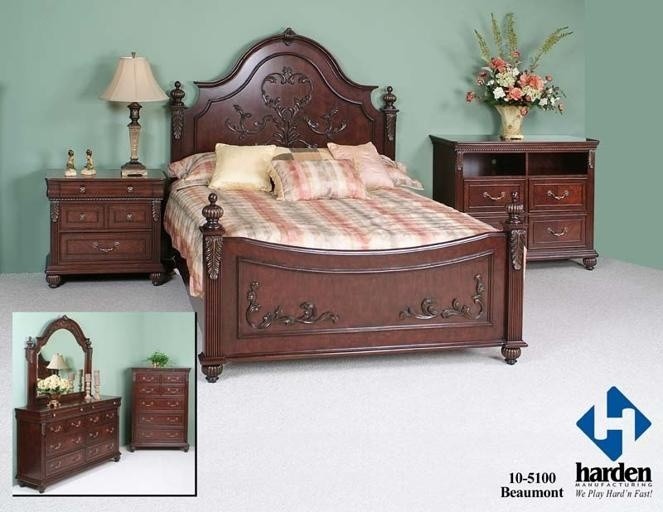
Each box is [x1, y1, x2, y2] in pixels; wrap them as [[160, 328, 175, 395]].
[[144, 350, 173, 368]]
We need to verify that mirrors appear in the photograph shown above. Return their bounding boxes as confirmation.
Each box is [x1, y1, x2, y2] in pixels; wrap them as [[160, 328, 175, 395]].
[[24, 314, 94, 410]]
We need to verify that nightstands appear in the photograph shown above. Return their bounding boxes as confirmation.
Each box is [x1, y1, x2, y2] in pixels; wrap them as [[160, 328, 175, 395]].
[[46, 168, 168, 290], [14, 394, 122, 494]]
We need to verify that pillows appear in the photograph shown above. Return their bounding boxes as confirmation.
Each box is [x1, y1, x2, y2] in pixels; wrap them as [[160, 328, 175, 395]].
[[207, 141, 278, 193], [170, 147, 425, 192], [326, 140, 395, 193], [269, 158, 374, 203]]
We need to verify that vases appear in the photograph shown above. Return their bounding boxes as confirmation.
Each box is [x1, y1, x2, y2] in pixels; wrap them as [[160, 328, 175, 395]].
[[494, 104, 529, 141]]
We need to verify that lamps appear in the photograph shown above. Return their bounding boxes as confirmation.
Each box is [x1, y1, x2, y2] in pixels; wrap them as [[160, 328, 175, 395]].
[[100, 50, 171, 178]]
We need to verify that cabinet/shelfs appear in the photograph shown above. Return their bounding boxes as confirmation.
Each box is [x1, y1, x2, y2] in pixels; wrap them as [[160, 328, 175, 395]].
[[429, 134, 600, 271], [167, 25, 529, 385], [129, 367, 192, 453]]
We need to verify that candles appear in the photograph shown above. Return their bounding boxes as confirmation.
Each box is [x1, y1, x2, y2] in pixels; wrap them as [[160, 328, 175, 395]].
[[84, 373, 92, 404], [93, 370, 101, 402]]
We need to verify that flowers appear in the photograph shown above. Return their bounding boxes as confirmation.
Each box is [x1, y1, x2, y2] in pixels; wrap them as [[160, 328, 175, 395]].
[[464, 11, 575, 118]]
